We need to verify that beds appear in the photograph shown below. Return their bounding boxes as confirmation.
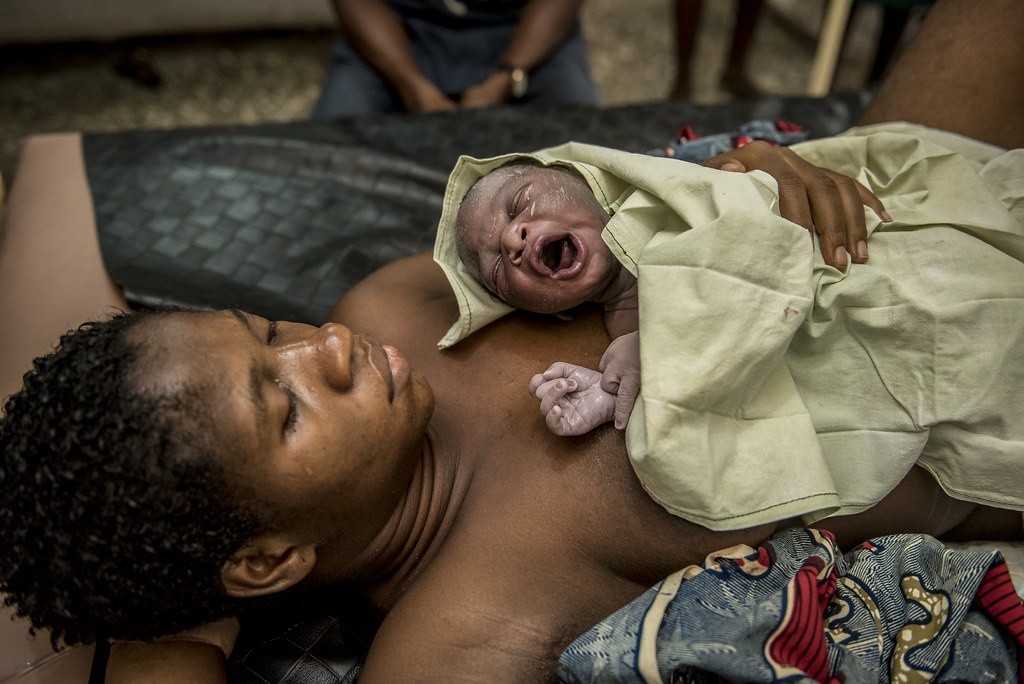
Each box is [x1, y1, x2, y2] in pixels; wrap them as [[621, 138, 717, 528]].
[[0, 100, 1023, 684]]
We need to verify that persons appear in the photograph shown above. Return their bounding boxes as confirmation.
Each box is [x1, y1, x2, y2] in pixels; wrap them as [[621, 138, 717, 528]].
[[666, 0, 764, 104], [310, 0, 597, 119], [0, 0, 1024, 684], [433, 121, 1024, 531]]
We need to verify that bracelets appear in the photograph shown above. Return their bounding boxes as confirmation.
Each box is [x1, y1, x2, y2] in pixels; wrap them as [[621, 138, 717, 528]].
[[496, 61, 528, 98]]
